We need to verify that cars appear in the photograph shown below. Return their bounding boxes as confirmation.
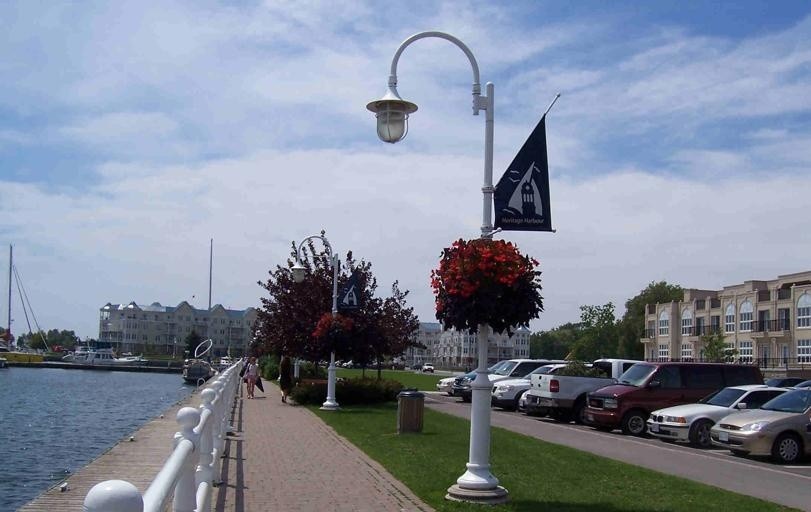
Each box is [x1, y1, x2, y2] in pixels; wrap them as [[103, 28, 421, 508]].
[[708, 386, 811, 465], [436, 357, 594, 413], [645, 383, 791, 447]]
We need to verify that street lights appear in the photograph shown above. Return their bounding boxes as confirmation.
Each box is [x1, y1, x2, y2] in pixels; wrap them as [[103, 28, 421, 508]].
[[290, 233, 346, 413], [365, 29, 512, 507]]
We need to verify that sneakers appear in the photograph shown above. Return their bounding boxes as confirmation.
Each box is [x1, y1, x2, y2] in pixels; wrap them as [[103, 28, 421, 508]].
[[282, 396, 284, 402], [248, 396, 254, 399]]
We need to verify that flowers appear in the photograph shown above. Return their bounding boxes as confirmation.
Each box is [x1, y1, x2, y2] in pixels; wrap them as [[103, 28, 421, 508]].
[[428, 234, 546, 339], [309, 312, 353, 342]]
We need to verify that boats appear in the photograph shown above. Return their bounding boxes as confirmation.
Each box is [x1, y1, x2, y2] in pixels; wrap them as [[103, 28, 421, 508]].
[[182, 358, 220, 383], [62, 347, 149, 367]]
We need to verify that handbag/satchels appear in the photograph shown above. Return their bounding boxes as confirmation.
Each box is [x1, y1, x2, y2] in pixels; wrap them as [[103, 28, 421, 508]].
[[244, 378, 248, 383]]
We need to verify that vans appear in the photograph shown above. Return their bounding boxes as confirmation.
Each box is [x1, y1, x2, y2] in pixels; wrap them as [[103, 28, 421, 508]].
[[585, 359, 765, 437]]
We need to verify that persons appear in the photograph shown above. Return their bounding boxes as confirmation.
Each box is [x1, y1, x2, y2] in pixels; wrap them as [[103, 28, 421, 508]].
[[279, 354, 296, 403], [239, 355, 252, 379], [244, 355, 262, 399]]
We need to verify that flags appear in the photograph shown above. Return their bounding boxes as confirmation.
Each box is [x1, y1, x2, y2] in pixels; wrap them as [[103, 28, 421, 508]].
[[494, 114, 553, 233]]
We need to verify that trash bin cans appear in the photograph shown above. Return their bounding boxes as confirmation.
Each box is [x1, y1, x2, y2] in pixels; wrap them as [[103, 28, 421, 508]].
[[397, 392, 425, 433]]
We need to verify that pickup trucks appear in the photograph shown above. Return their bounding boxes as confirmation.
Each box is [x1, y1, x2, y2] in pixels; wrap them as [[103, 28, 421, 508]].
[[526, 358, 648, 425]]
